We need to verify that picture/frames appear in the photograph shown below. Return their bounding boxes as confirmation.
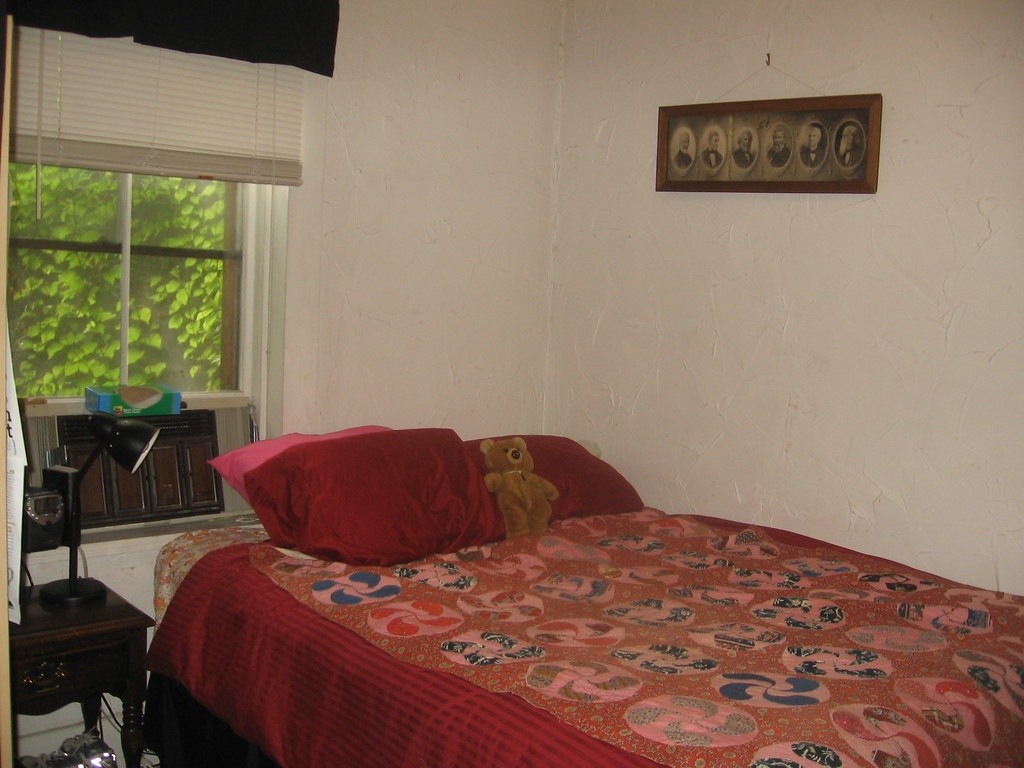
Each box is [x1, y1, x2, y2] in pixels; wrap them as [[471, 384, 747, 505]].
[[655, 93, 882, 194]]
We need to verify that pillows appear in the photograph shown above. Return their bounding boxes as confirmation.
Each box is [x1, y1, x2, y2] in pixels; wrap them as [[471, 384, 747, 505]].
[[463, 434, 644, 521], [244, 427, 505, 565], [207, 426, 394, 511]]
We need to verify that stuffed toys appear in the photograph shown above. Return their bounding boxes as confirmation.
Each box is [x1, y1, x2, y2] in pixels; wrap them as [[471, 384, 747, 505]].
[[481, 437, 559, 539]]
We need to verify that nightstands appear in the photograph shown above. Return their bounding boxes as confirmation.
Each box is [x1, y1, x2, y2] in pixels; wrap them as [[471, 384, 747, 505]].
[[9, 575, 157, 768]]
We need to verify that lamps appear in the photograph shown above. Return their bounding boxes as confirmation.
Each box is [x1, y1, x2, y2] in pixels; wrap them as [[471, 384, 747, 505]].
[[38, 409, 161, 611]]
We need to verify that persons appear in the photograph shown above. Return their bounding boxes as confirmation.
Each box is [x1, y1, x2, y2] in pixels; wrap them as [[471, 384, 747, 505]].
[[800, 127, 825, 168], [836, 126, 863, 167], [701, 131, 723, 169], [673, 133, 693, 170], [767, 129, 790, 167], [732, 130, 755, 169]]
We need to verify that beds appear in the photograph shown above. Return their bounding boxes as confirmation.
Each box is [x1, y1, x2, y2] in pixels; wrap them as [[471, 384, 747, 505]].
[[138, 507, 1024, 768]]
